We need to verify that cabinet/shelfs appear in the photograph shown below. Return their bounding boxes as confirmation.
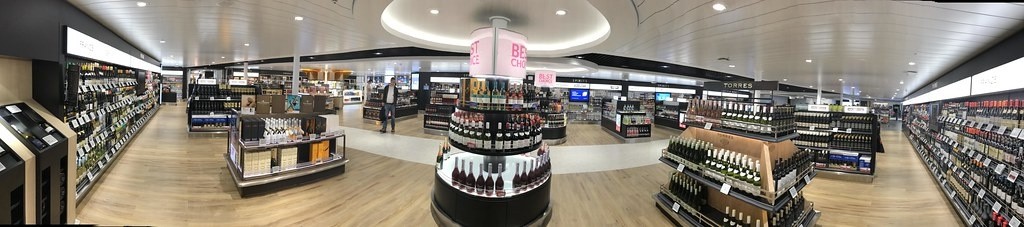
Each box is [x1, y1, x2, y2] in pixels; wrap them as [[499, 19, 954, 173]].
[[186, 70, 361, 198], [423, 82, 568, 227], [568, 87, 651, 146], [362, 86, 418, 126], [902, 88, 1024, 227], [651, 91, 902, 227], [64, 61, 184, 198]]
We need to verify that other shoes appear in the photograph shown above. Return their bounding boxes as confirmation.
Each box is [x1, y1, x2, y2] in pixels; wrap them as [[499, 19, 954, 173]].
[[391, 128, 395, 132], [380, 127, 386, 133]]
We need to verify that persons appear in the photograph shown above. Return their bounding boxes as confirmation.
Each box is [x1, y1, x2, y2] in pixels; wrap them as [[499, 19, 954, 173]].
[[379, 77, 398, 133], [367, 79, 373, 100]]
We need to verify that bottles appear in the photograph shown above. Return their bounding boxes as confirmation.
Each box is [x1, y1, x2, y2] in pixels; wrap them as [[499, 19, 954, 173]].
[[623, 97, 873, 227], [907, 99, 1024, 227], [192, 86, 256, 128], [67, 61, 158, 185], [17, 128, 46, 149], [32, 117, 55, 134], [271, 157, 277, 167], [426, 84, 601, 195], [262, 118, 302, 144]]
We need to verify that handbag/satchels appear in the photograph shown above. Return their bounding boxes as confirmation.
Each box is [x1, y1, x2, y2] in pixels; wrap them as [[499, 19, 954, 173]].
[[379, 106, 386, 123]]
